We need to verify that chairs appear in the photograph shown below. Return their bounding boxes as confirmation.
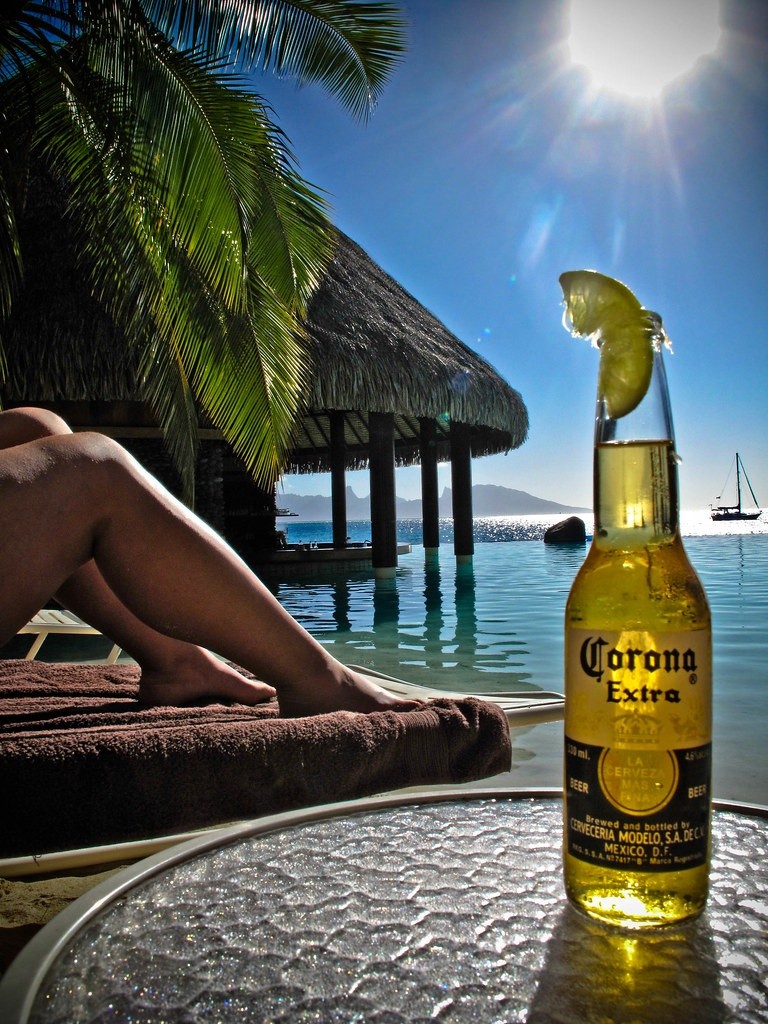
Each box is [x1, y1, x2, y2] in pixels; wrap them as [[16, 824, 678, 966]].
[[15, 608, 124, 664], [1, 660, 513, 881]]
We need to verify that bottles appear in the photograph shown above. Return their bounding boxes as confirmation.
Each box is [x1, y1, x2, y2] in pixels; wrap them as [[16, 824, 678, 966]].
[[275, 507, 290, 515], [562, 309, 713, 934]]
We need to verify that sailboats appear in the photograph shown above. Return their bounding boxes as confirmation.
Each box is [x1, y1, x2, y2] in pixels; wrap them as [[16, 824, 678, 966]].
[[709, 452, 763, 521]]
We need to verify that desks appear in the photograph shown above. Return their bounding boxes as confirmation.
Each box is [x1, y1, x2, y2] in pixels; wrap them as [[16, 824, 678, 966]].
[[0, 786, 767, 1024]]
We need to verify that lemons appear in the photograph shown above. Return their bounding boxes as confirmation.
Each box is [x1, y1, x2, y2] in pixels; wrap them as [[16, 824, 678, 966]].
[[557, 272, 653, 421]]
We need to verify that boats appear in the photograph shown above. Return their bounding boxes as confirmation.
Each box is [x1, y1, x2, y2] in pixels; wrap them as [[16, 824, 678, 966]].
[[277, 528, 412, 564], [275, 508, 299, 524]]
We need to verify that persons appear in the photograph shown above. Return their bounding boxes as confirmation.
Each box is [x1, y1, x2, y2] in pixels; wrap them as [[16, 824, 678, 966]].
[[0, 407, 420, 719]]
